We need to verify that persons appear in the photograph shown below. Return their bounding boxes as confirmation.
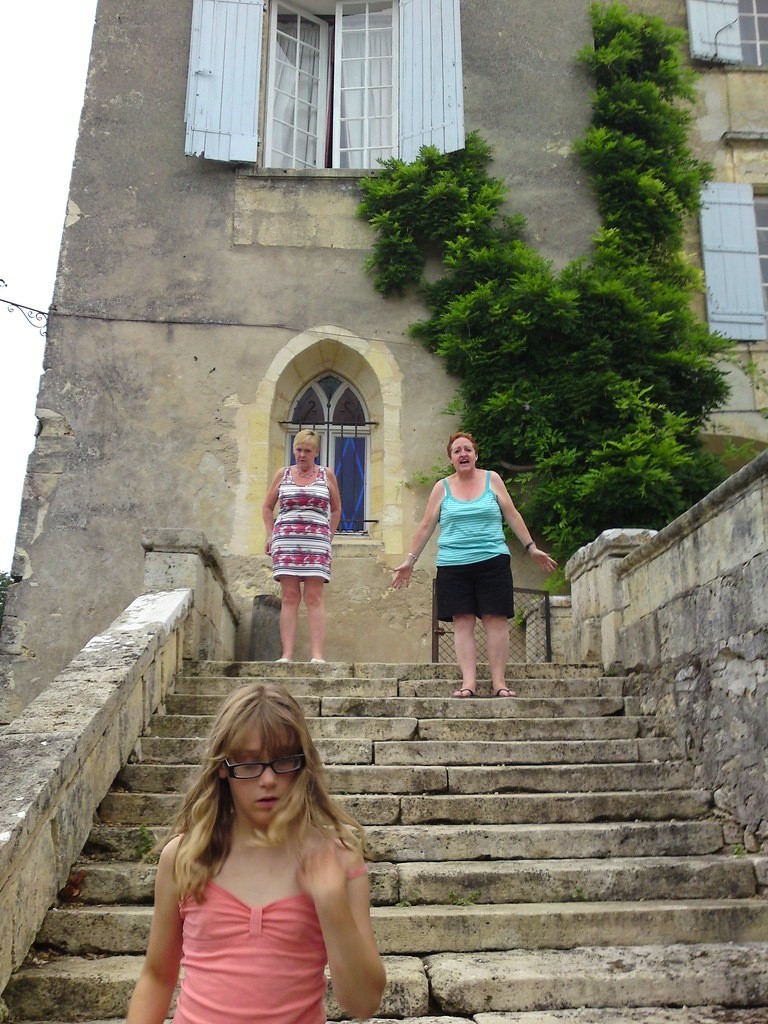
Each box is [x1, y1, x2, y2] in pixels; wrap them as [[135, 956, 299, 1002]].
[[263, 430, 342, 662], [125, 684, 386, 1024], [391, 432, 557, 698]]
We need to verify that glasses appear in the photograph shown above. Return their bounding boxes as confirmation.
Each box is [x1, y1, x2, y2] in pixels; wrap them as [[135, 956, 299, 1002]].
[[224, 754, 305, 779]]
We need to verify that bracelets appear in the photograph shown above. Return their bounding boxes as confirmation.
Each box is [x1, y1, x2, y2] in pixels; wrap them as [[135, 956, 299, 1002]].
[[526, 541, 534, 551], [408, 552, 418, 561]]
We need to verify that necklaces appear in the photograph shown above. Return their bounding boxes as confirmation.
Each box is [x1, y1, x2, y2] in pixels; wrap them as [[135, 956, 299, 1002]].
[[296, 464, 315, 479]]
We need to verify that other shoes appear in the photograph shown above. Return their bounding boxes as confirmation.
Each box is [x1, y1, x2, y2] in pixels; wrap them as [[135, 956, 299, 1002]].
[[275, 657, 292, 663], [311, 658, 325, 663]]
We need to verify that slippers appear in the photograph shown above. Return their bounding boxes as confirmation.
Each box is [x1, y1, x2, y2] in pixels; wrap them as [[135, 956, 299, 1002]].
[[451, 689, 479, 698], [490, 689, 518, 697]]
